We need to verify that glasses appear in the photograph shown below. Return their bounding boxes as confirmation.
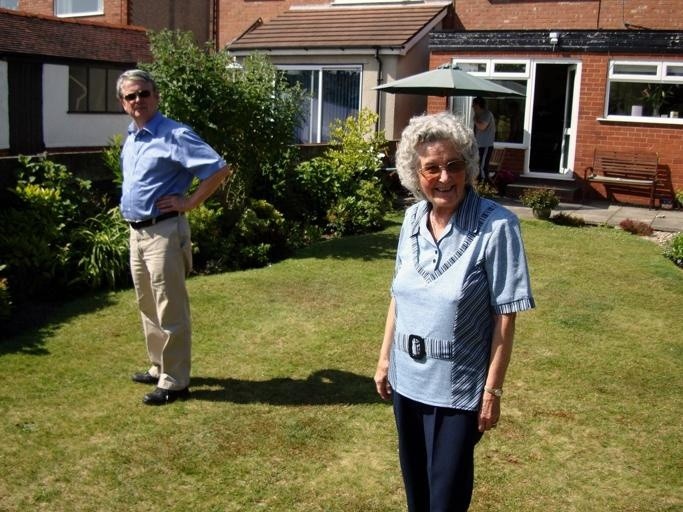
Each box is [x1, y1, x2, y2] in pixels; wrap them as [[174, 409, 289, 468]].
[[122, 88, 151, 100], [418, 158, 468, 176]]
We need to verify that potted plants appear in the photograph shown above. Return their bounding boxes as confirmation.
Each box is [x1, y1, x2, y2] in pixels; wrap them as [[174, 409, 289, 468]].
[[519, 186, 561, 220]]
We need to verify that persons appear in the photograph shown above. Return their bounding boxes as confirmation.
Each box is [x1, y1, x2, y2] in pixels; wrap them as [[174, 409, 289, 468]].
[[471, 98, 496, 185], [372, 112, 535, 512], [112, 70, 231, 405]]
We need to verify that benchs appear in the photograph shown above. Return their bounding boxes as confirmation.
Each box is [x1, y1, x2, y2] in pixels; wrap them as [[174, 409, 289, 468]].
[[580, 146, 659, 210]]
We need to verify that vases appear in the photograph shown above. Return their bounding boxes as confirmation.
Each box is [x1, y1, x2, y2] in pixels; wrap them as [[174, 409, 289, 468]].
[[652, 101, 663, 116]]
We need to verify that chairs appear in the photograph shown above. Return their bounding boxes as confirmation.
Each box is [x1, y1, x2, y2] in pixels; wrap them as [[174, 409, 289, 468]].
[[488, 148, 505, 182]]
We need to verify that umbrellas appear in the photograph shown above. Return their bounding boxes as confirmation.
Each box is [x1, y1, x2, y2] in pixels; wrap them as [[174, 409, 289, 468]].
[[371, 64, 527, 113]]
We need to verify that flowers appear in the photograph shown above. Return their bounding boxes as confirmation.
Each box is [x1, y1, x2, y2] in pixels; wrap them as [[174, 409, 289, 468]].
[[631, 83, 675, 107]]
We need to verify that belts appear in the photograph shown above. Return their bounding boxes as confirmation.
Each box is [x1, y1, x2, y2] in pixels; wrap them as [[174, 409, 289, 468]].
[[130, 209, 185, 230]]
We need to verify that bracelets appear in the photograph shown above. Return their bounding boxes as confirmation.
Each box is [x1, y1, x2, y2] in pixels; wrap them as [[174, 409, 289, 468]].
[[484, 386, 505, 396]]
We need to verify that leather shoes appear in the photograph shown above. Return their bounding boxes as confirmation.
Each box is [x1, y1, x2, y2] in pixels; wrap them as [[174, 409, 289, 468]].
[[132, 372, 159, 384], [142, 387, 188, 405]]
